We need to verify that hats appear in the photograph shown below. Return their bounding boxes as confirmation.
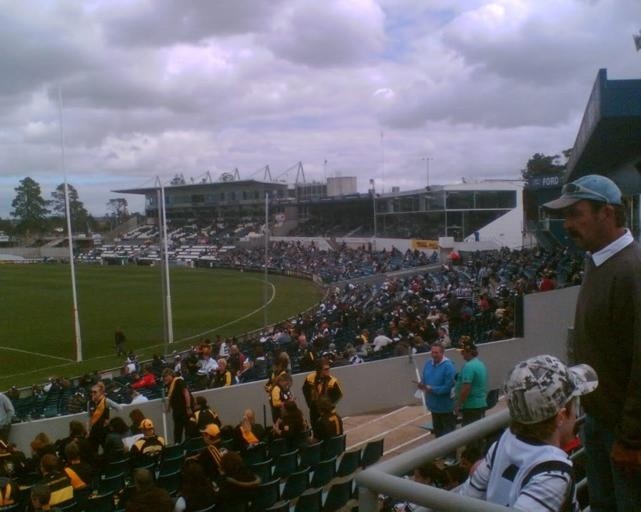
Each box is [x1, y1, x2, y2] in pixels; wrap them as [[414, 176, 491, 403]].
[[505, 353, 600, 424], [200, 423, 222, 438], [463, 341, 478, 351], [138, 419, 153, 430], [542, 173, 625, 209]]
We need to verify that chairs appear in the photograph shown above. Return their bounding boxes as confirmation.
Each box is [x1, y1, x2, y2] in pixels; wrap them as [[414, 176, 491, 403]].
[[408, 310, 524, 355], [298, 310, 408, 373], [417, 387, 592, 512], [98, 366, 210, 406], [0, 424, 384, 512], [79, 209, 327, 283], [326, 207, 590, 310], [10, 387, 98, 423], [238, 317, 298, 384]]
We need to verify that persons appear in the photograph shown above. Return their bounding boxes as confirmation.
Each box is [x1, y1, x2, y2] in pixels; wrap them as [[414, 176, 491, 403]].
[[542, 172, 641, 512], [299, 219, 591, 317], [43, 219, 301, 277]]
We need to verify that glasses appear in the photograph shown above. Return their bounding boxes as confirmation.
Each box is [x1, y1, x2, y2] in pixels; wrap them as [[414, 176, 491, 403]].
[[90, 390, 96, 392], [561, 184, 610, 203]]
